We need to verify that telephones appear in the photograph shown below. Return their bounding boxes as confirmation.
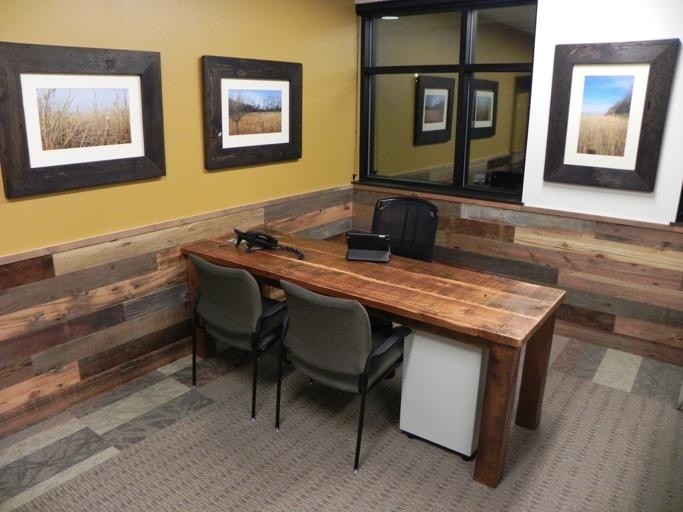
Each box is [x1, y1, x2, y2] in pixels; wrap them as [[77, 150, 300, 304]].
[[234, 228, 278, 253]]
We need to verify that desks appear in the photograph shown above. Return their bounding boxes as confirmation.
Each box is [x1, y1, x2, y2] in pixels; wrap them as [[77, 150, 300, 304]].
[[184, 227, 567, 489]]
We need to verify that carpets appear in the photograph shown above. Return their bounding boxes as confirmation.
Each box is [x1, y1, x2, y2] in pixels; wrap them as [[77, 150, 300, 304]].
[[16, 367, 683, 512]]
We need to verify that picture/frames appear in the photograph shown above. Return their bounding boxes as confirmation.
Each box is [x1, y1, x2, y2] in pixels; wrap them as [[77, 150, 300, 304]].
[[0, 42, 170, 201], [198, 51, 305, 177], [413, 75, 500, 147], [542, 36, 681, 193]]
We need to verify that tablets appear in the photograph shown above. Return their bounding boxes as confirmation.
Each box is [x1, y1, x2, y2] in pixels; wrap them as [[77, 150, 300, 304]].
[[345, 233, 393, 251]]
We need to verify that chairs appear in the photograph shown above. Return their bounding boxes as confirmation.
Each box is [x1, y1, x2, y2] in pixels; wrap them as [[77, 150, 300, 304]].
[[370, 198, 439, 264], [186, 250, 286, 424], [274, 276, 414, 475]]
